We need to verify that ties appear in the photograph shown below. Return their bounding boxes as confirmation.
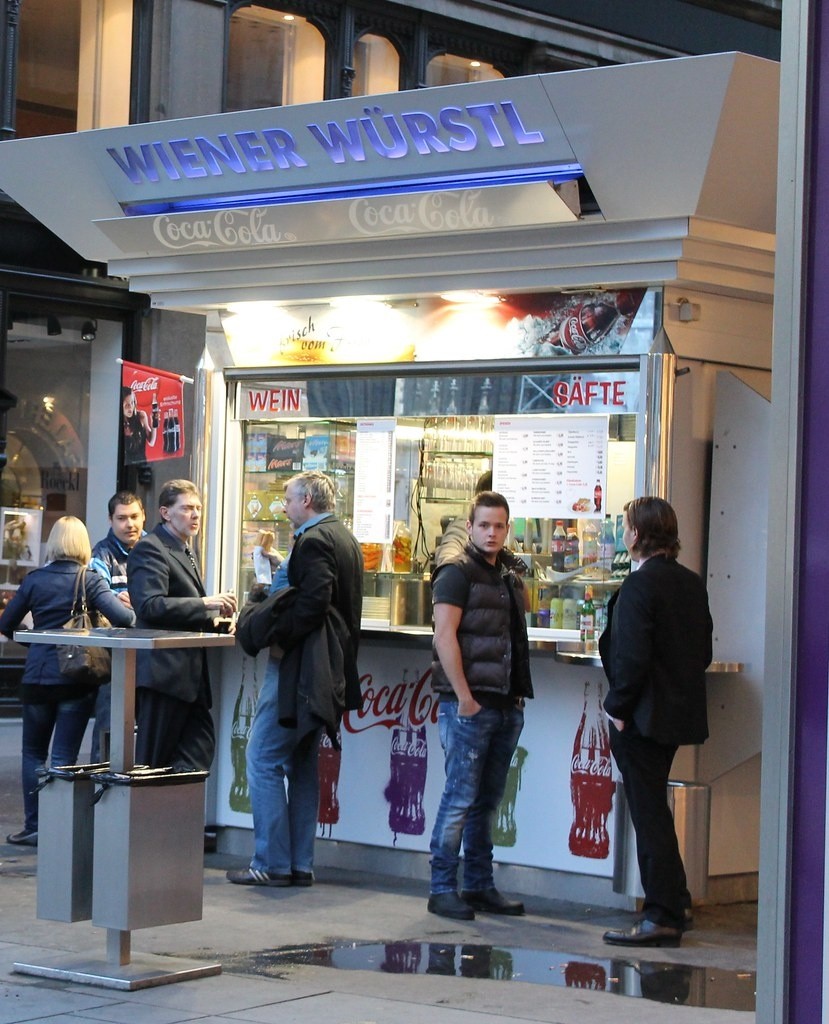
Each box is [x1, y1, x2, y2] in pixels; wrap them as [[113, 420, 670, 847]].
[[185, 548, 196, 572]]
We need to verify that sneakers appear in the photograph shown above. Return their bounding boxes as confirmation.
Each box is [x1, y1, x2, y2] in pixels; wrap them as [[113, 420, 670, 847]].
[[227, 867, 290, 887], [290, 870, 313, 886]]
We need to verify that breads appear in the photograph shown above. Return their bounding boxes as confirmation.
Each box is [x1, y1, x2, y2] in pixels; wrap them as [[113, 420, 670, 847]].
[[254, 529, 275, 551]]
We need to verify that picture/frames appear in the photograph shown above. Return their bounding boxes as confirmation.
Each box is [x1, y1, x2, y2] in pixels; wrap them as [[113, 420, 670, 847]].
[[0, 506, 44, 567]]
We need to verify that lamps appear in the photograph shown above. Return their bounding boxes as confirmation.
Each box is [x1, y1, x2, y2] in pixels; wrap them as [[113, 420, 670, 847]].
[[47, 314, 61, 336], [81, 315, 98, 341]]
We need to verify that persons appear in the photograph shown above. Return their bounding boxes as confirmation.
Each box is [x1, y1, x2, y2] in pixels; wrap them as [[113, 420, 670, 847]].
[[126, 480, 239, 770], [226, 472, 364, 886], [123, 387, 160, 463], [437, 469, 493, 565], [597, 497, 711, 946], [1, 514, 138, 847], [87, 490, 149, 764], [427, 490, 534, 920]]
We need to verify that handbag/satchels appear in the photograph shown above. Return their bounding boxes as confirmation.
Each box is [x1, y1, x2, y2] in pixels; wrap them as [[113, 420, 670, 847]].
[[56, 613, 111, 684]]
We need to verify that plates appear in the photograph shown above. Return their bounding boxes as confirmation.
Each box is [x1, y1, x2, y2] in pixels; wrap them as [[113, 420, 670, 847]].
[[361, 597, 390, 619]]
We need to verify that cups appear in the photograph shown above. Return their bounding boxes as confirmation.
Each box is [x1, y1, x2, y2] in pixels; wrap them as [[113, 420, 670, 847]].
[[549, 596, 562, 628], [562, 598, 577, 630]]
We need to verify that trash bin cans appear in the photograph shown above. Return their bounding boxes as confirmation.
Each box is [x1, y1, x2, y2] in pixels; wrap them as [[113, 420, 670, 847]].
[[34, 761, 149, 923], [613, 779, 711, 901], [91, 767, 210, 930]]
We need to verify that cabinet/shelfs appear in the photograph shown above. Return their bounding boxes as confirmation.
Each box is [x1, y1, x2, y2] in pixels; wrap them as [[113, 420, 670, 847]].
[[240, 420, 354, 574], [418, 450, 494, 503]]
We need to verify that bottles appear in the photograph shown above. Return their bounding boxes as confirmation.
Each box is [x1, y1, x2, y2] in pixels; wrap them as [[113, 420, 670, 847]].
[[358, 523, 383, 573], [551, 520, 567, 572], [582, 520, 599, 575], [579, 585, 595, 640], [565, 527, 579, 573], [537, 583, 552, 628], [392, 520, 412, 573], [163, 408, 181, 454], [599, 513, 616, 572], [151, 393, 159, 428]]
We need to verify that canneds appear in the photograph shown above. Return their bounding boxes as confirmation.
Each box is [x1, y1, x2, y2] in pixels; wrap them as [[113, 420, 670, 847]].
[[549, 597, 604, 631]]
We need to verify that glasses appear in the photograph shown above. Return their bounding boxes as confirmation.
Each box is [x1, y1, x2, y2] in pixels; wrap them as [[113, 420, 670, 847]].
[[281, 493, 300, 507]]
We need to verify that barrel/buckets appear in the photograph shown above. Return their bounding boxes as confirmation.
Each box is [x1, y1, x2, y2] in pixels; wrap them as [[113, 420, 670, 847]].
[[390, 578, 433, 626]]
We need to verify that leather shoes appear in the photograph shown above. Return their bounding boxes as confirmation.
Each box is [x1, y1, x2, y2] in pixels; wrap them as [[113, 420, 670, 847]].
[[603, 917, 681, 947], [428, 891, 474, 920], [7, 829, 38, 847], [462, 887, 524, 916], [633, 908, 694, 930]]
